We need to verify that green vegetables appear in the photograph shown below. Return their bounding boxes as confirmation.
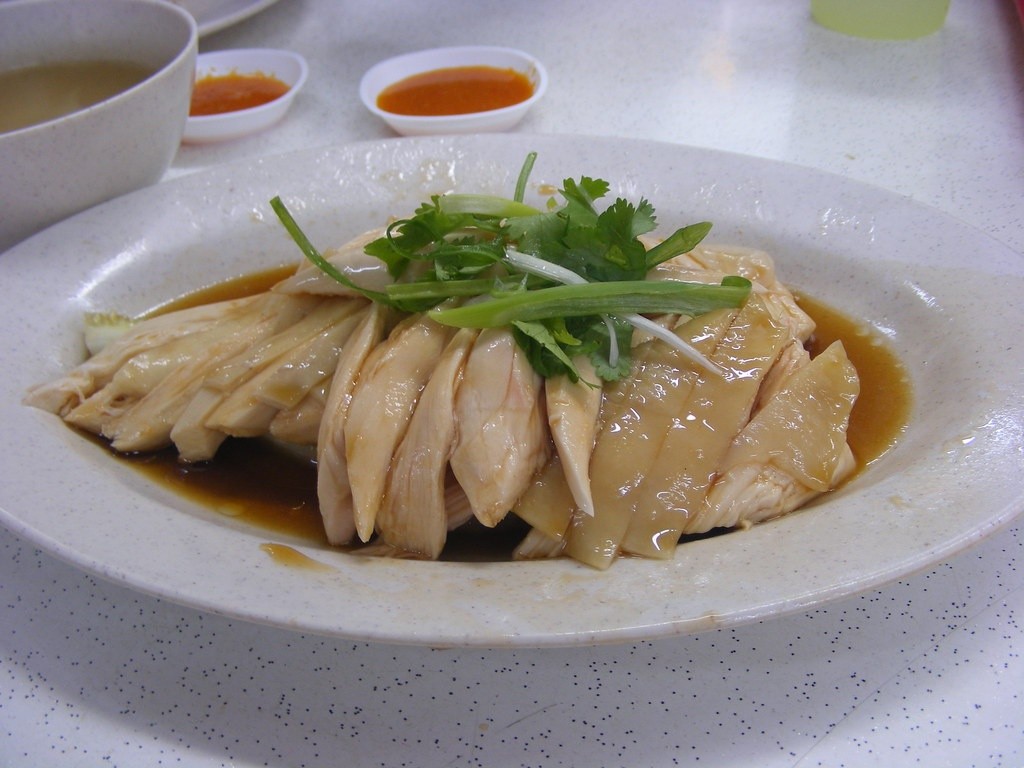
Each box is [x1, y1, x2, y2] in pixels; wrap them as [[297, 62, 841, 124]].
[[272, 153, 752, 383]]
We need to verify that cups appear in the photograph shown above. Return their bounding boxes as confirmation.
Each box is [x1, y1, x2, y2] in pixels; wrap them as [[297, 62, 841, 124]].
[[810, 0, 952, 40]]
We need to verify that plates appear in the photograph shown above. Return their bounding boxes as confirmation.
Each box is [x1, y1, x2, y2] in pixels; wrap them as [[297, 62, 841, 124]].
[[0, 132, 1024, 649], [357, 44, 550, 136], [180, 48, 309, 144]]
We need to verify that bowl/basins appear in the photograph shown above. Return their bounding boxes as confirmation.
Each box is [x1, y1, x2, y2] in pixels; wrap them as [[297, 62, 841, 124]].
[[0, 0, 200, 248]]
